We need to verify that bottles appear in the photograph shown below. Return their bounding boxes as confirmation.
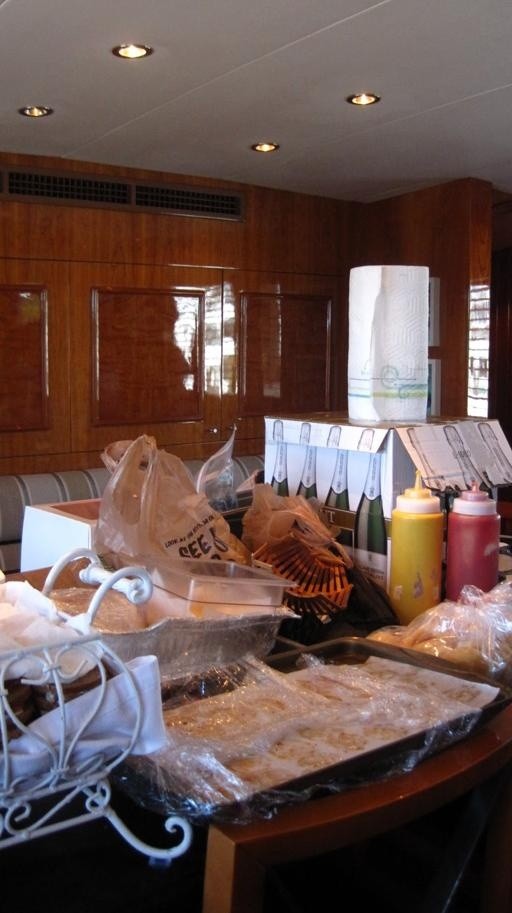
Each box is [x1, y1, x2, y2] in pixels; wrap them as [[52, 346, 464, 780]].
[[404, 421, 512, 502], [271, 443, 291, 498], [445, 476, 499, 605], [320, 447, 354, 550], [353, 451, 388, 590], [295, 445, 322, 501], [388, 470, 449, 624]]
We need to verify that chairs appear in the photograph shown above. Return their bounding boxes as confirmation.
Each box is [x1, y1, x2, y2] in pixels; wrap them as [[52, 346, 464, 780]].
[[202, 703, 512, 912]]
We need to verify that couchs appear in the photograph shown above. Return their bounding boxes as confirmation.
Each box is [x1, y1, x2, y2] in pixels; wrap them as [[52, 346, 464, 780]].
[[0, 455, 265, 574]]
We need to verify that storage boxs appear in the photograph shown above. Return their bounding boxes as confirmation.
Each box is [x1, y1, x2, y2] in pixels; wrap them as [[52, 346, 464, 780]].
[[264, 414, 511, 603]]
